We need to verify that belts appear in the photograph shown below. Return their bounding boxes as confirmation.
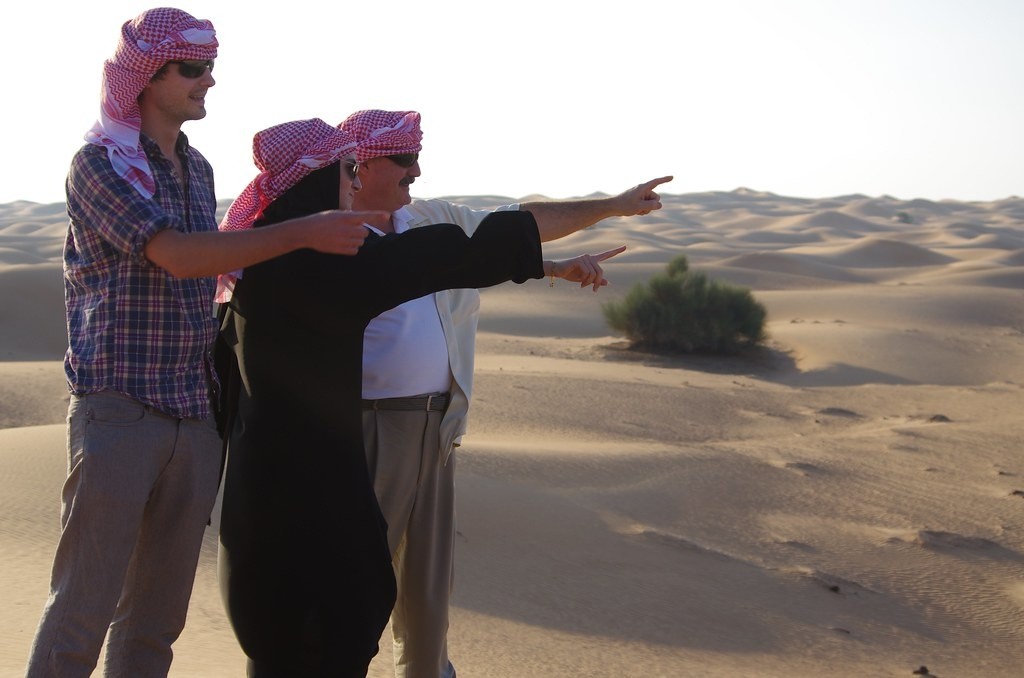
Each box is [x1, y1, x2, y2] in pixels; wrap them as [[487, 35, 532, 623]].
[[362, 391, 450, 412]]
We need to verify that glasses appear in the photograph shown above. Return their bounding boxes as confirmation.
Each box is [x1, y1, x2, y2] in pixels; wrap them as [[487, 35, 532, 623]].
[[169, 59, 214, 78], [382, 153, 419, 167], [340, 160, 360, 177]]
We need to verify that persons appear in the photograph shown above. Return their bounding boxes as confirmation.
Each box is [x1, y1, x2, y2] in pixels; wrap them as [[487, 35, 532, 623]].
[[339, 109, 674, 678], [217, 114, 632, 678], [22, 7, 391, 677]]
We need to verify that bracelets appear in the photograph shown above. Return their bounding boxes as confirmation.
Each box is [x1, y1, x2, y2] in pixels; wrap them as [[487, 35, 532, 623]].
[[548, 260, 557, 288]]
